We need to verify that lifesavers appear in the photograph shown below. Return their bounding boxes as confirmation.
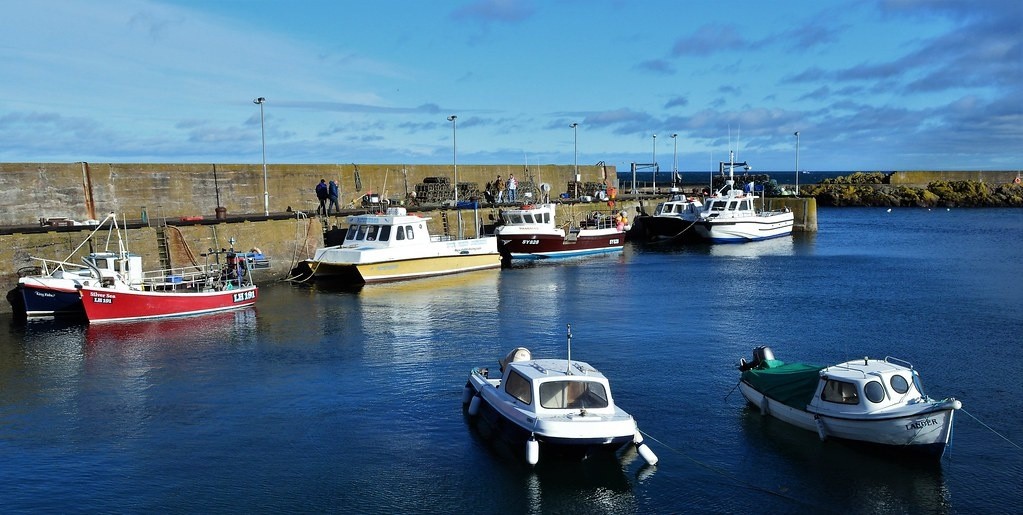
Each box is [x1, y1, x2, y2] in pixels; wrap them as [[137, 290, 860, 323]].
[[249, 247, 262, 254]]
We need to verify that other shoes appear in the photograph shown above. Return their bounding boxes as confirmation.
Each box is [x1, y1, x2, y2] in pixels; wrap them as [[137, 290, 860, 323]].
[[336, 212, 341, 216], [322, 212, 326, 217], [317, 210, 320, 214], [329, 212, 334, 216]]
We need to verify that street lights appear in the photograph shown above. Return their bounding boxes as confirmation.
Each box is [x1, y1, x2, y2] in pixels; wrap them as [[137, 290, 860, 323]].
[[569, 122, 578, 198], [653, 135, 656, 193], [446, 115, 458, 207], [795, 132, 800, 196], [254, 97, 269, 217], [671, 133, 678, 188]]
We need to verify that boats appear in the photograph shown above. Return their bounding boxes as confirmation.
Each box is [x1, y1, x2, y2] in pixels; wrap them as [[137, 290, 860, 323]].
[[461, 323, 637, 464], [735, 344, 961, 458], [358, 269, 499, 337], [632, 193, 704, 241], [710, 234, 795, 257], [297, 206, 501, 282], [491, 200, 627, 257], [6, 212, 259, 324], [701, 121, 794, 241]]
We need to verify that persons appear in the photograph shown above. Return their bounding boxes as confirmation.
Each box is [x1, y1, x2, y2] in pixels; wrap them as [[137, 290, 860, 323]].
[[328, 179, 341, 213], [496, 175, 505, 204], [507, 174, 517, 202], [316, 179, 327, 215]]
